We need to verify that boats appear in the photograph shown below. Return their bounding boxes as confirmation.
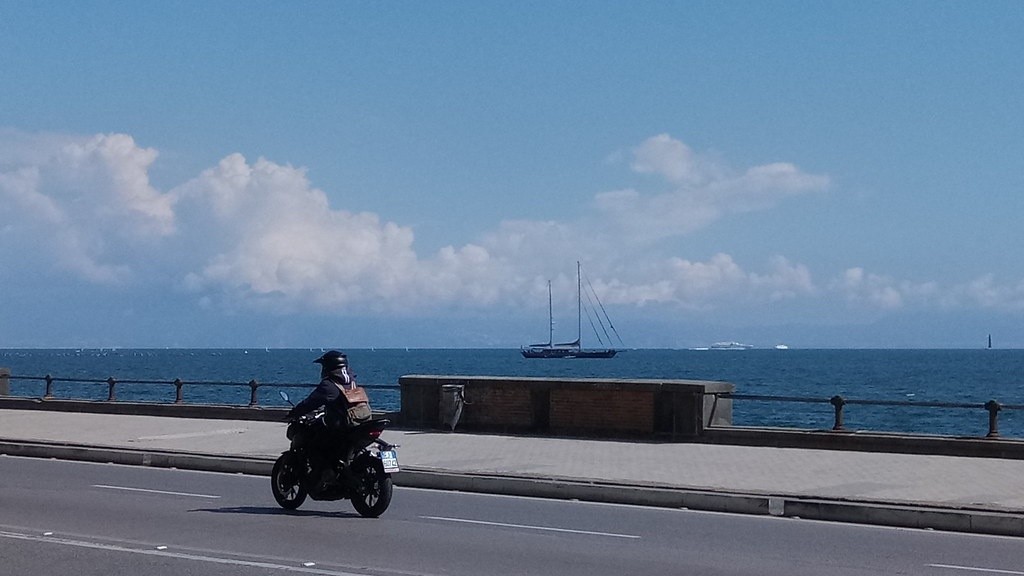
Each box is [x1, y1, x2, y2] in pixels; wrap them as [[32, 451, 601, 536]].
[[687, 342, 754, 351], [521, 261, 617, 359], [773, 344, 789, 350]]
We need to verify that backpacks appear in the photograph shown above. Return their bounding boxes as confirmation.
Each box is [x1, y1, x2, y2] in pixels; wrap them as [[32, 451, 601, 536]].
[[331, 378, 373, 429]]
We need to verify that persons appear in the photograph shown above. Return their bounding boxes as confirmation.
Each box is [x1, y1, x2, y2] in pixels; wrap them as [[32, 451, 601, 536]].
[[289, 350, 372, 493]]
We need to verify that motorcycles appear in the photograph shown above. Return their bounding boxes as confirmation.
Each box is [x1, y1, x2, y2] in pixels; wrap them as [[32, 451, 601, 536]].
[[271, 390, 400, 517]]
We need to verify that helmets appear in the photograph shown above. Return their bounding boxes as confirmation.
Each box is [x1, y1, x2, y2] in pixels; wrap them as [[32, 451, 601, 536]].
[[312, 350, 348, 373]]
